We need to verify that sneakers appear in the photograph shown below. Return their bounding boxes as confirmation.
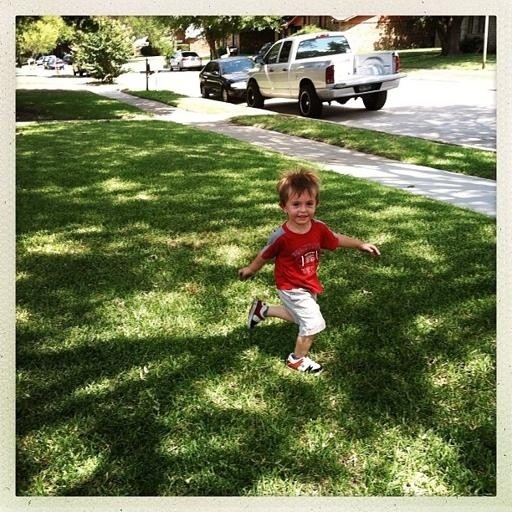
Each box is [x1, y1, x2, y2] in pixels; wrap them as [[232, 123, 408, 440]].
[[247, 299, 268, 330], [287, 352, 323, 373]]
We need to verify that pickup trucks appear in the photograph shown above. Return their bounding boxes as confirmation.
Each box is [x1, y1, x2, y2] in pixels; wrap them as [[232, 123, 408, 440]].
[[247, 31, 406, 117]]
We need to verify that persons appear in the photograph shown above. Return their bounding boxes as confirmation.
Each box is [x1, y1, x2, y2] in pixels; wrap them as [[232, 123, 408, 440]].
[[237, 166, 379, 374], [16, 57, 22, 68]]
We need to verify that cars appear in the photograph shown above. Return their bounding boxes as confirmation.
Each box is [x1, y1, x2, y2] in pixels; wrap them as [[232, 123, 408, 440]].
[[37, 54, 86, 77], [168, 51, 202, 71], [199, 56, 257, 103]]
